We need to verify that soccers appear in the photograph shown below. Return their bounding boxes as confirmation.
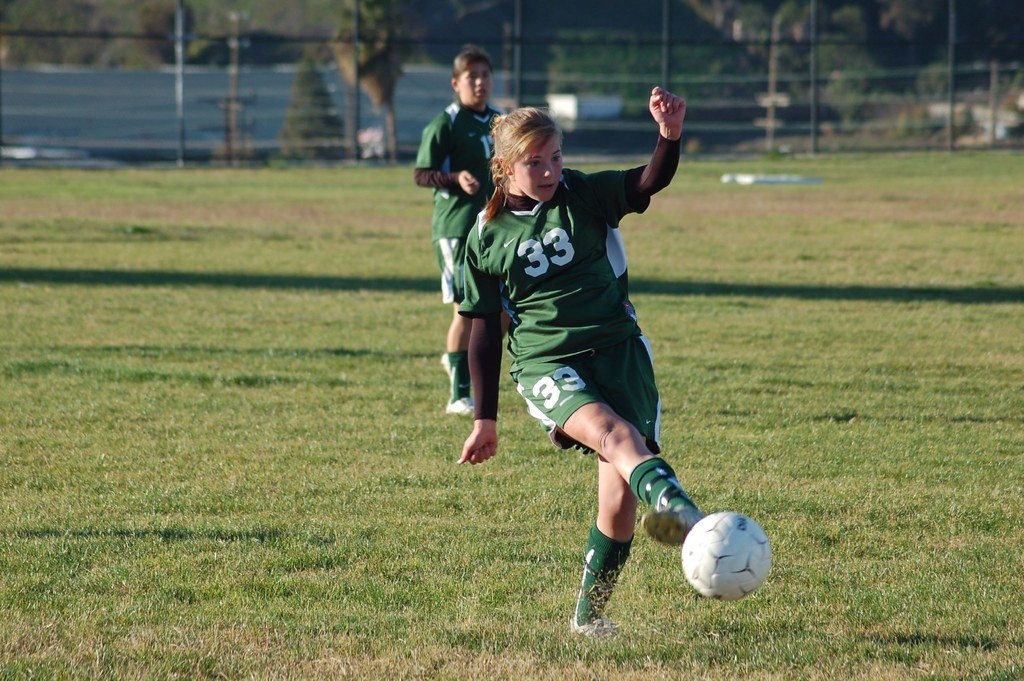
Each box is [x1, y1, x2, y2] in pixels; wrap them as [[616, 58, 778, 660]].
[[680, 511, 772, 600]]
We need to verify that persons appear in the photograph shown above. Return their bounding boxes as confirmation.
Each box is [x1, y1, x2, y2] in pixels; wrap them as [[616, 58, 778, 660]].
[[412, 44, 513, 419], [456, 84, 710, 643]]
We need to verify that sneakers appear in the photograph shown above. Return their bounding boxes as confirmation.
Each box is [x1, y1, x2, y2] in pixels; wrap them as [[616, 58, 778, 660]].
[[570, 617, 615, 639], [446, 397, 476, 417], [642, 510, 710, 546]]
